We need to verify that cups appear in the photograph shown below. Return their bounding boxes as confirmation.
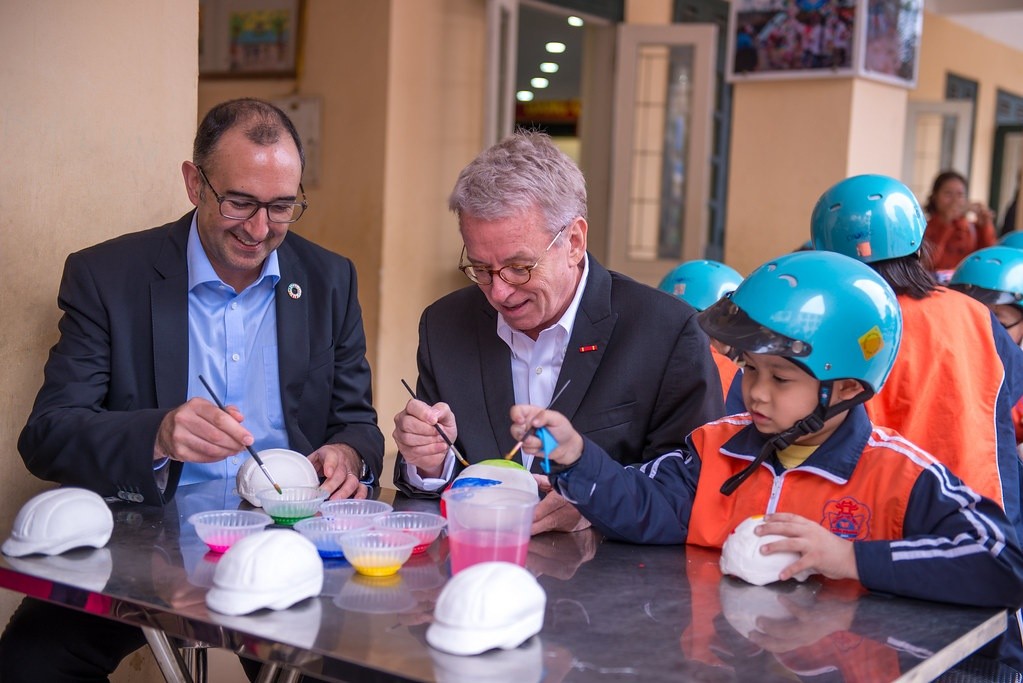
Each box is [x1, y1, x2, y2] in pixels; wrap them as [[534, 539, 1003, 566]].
[[442, 487, 539, 577]]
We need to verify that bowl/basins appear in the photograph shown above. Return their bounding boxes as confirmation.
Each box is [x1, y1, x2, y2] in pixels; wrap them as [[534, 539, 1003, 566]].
[[293, 517, 373, 559], [400, 556, 445, 591], [188, 510, 275, 553], [187, 548, 224, 588], [375, 511, 447, 553], [340, 530, 419, 576], [333, 577, 417, 615], [319, 499, 393, 524], [256, 486, 330, 525]]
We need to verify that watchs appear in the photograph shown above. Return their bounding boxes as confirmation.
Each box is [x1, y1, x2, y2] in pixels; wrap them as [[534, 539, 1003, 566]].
[[361, 459, 369, 479]]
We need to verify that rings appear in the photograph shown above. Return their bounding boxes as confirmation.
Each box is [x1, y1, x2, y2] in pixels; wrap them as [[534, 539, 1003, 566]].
[[347, 473, 359, 479]]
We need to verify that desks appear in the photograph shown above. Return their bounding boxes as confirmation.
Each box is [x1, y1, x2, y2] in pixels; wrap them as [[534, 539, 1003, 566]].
[[0, 475, 1008, 683]]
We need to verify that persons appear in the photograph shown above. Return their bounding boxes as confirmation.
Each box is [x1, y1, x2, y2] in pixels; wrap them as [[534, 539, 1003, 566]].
[[390, 132, 726, 537], [659, 170, 1023, 683], [0, 97, 385, 683], [643, 543, 1005, 683], [510, 250, 1023, 617], [734, 8, 850, 72]]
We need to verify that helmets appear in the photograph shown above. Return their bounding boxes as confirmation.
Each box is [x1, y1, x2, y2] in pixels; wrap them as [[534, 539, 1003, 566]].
[[697, 251, 903, 394], [1001, 232, 1023, 249], [656, 260, 745, 313], [946, 245, 1023, 307], [810, 174, 928, 263]]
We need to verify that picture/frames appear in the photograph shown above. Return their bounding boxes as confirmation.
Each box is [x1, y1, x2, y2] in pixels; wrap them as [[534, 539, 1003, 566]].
[[198, 0, 302, 80]]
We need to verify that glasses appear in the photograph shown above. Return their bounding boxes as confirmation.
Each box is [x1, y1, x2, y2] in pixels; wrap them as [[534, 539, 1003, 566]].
[[458, 217, 575, 286], [196, 165, 307, 223]]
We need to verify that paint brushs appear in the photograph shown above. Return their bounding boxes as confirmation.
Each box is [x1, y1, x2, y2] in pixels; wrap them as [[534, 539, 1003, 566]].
[[199, 374, 282, 494], [504, 379, 571, 461], [401, 378, 470, 467]]
